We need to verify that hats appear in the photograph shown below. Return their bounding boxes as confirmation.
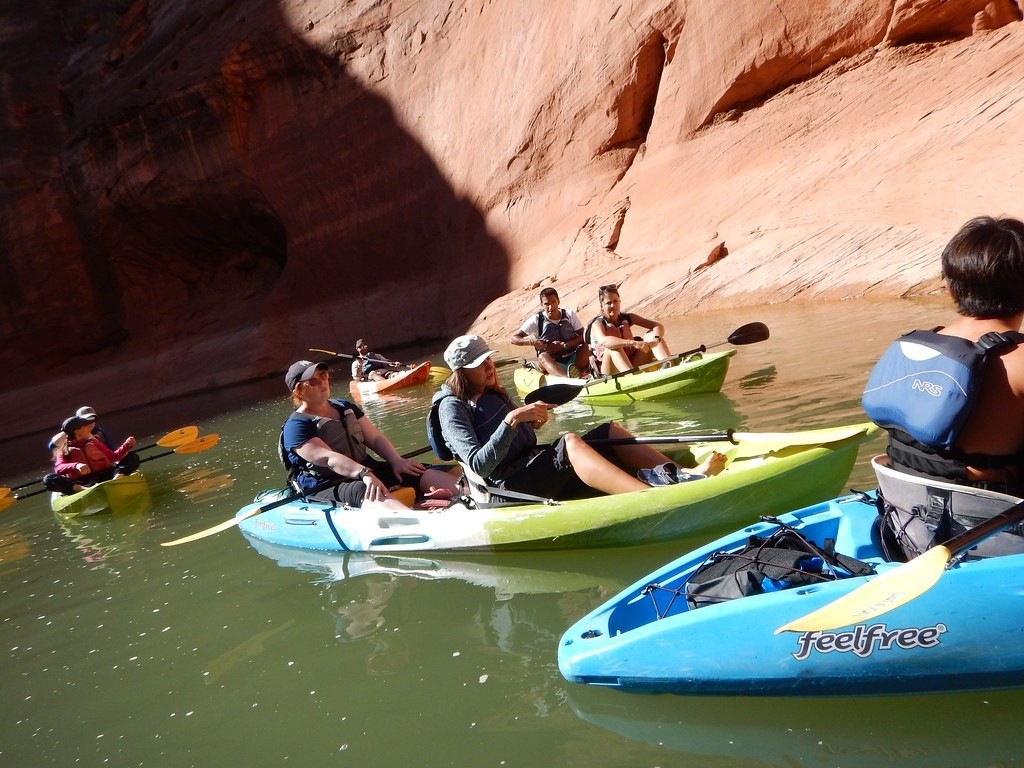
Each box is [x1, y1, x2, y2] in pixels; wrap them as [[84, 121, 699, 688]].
[[76, 406, 99, 419], [61, 416, 97, 434], [356, 340, 368, 348], [284, 360, 328, 392], [443, 335, 500, 372]]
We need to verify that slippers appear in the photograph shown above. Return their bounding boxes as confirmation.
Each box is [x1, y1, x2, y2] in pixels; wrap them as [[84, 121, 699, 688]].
[[638, 463, 710, 489]]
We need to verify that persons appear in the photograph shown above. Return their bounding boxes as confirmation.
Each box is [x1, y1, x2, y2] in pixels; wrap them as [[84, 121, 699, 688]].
[[510, 288, 594, 379], [277, 360, 469, 511], [584, 283, 673, 378], [48, 405, 117, 452], [42, 415, 140, 497], [350, 339, 418, 382], [432, 334, 727, 502], [862, 215, 1024, 561]]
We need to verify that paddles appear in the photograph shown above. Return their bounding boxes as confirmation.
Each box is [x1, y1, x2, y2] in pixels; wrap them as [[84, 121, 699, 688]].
[[157, 445, 433, 548], [524, 321, 770, 411], [771, 501, 1024, 637], [536, 422, 869, 446], [0, 424, 220, 512], [308, 348, 451, 376]]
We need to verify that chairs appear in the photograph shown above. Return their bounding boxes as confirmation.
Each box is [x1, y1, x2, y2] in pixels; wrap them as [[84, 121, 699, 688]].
[[870, 453, 1024, 567], [457, 462, 489, 508]]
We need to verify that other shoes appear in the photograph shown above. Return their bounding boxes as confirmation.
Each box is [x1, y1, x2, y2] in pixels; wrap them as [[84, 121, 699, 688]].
[[683, 353, 701, 363]]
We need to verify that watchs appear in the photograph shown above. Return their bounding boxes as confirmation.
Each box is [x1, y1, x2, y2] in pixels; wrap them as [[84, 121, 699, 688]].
[[359, 466, 373, 479], [653, 335, 661, 342], [563, 341, 569, 350]]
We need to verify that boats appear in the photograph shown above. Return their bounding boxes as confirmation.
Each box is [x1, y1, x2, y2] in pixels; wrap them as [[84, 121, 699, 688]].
[[512, 348, 740, 403], [553, 483, 1024, 698], [348, 361, 433, 396], [234, 421, 887, 552], [51, 463, 150, 518]]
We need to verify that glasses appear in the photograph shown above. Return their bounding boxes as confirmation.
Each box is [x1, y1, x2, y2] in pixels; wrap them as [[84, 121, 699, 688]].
[[358, 344, 367, 350], [301, 370, 328, 390], [599, 284, 616, 294]]
